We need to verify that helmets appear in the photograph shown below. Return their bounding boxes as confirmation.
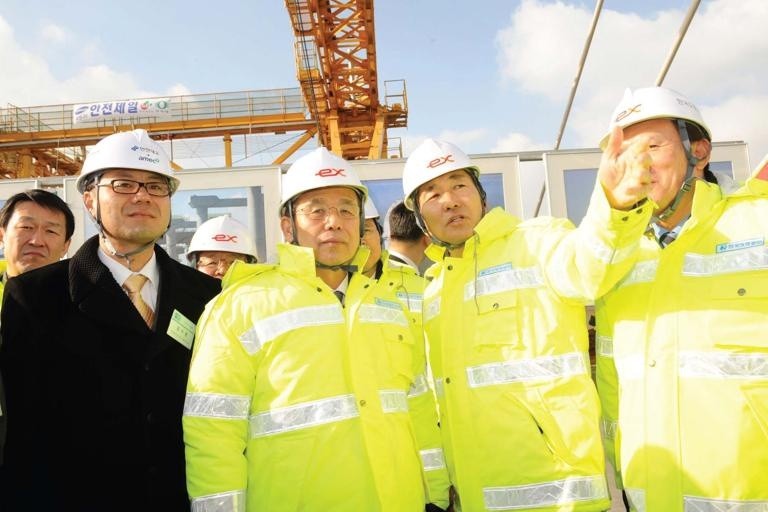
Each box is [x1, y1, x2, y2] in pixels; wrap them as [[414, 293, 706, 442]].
[[402, 138, 480, 211], [186, 214, 259, 263], [600, 86, 712, 152], [277, 147, 380, 219], [75, 128, 181, 196]]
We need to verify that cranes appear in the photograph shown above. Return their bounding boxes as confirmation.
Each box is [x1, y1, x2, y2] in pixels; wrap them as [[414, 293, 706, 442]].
[[1, 0, 408, 181]]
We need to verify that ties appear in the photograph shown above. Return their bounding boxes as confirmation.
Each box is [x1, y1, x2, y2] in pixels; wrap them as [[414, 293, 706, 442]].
[[122, 273, 155, 330]]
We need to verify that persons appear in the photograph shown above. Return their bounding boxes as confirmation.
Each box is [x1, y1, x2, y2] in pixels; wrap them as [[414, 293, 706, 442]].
[[0, 85, 768, 512]]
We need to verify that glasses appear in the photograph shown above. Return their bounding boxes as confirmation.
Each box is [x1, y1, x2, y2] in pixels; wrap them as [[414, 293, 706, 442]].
[[90, 179, 171, 196]]
